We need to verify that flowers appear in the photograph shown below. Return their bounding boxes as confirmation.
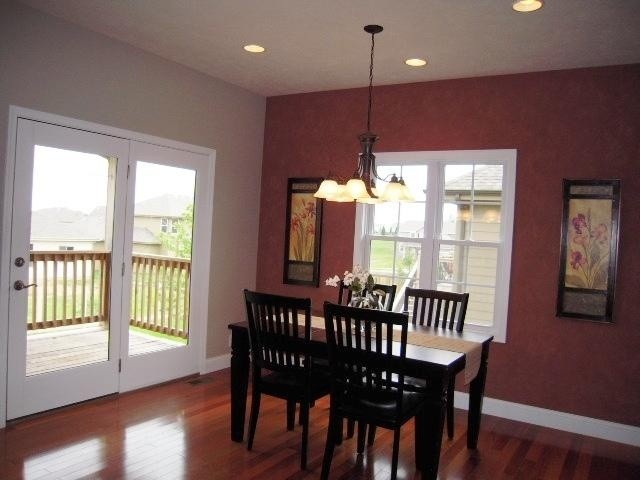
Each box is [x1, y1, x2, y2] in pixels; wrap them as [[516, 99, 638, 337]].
[[324, 265, 386, 309]]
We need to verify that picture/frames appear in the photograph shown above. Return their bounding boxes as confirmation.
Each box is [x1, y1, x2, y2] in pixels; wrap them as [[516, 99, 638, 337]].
[[283, 178, 323, 288], [556, 178, 622, 326]]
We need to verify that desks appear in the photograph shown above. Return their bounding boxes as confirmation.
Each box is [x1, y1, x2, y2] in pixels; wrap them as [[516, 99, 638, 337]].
[[228, 310, 494, 449]]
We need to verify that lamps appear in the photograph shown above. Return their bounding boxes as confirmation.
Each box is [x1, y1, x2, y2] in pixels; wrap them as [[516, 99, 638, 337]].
[[314, 25, 410, 205]]
